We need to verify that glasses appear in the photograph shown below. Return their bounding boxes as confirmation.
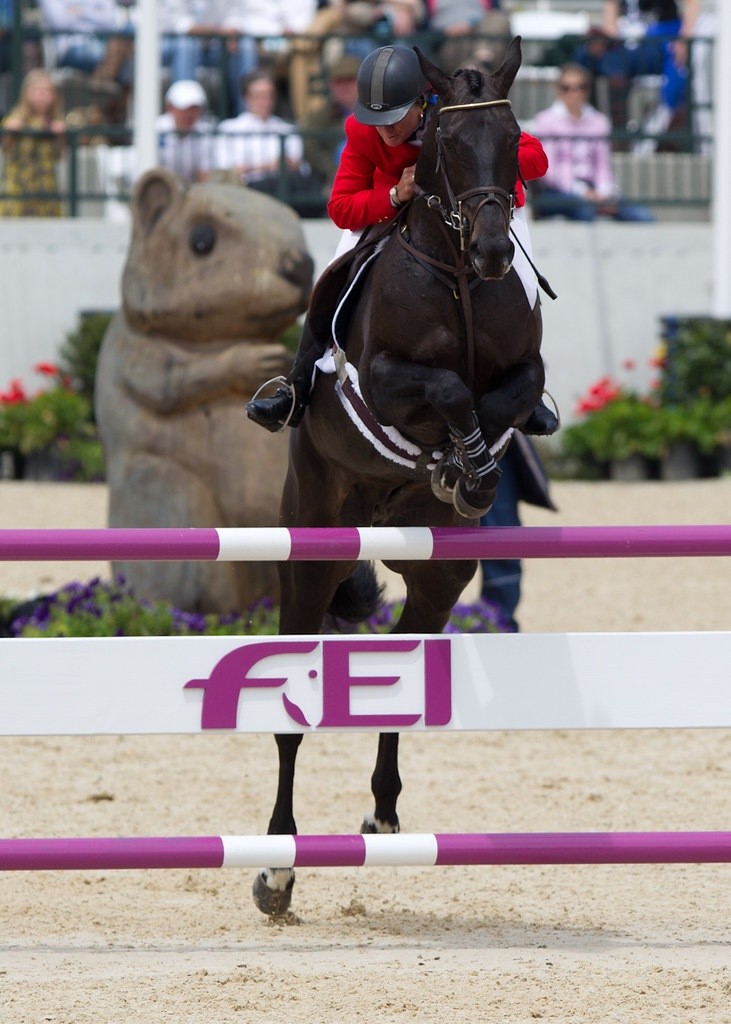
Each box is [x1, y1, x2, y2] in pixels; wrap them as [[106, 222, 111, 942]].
[[557, 81, 589, 93]]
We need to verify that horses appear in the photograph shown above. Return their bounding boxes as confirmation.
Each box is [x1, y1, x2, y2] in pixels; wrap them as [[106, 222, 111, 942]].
[[250, 34, 560, 921]]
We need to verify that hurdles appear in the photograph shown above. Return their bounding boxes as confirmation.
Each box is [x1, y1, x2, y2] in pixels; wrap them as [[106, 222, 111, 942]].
[[0, 523, 730, 873]]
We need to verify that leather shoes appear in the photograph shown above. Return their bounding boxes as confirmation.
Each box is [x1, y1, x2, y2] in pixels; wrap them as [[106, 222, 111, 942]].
[[519, 398, 559, 436], [243, 385, 304, 429]]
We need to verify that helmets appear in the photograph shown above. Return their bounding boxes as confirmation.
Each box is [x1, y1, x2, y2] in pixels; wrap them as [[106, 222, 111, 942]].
[[353, 44, 425, 126]]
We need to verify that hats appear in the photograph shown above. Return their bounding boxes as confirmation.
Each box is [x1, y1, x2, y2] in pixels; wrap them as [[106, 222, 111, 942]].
[[166, 79, 208, 111]]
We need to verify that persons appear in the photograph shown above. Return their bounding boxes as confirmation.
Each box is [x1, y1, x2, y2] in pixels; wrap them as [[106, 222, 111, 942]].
[[244, 47, 559, 435], [0, 0, 731, 223]]
